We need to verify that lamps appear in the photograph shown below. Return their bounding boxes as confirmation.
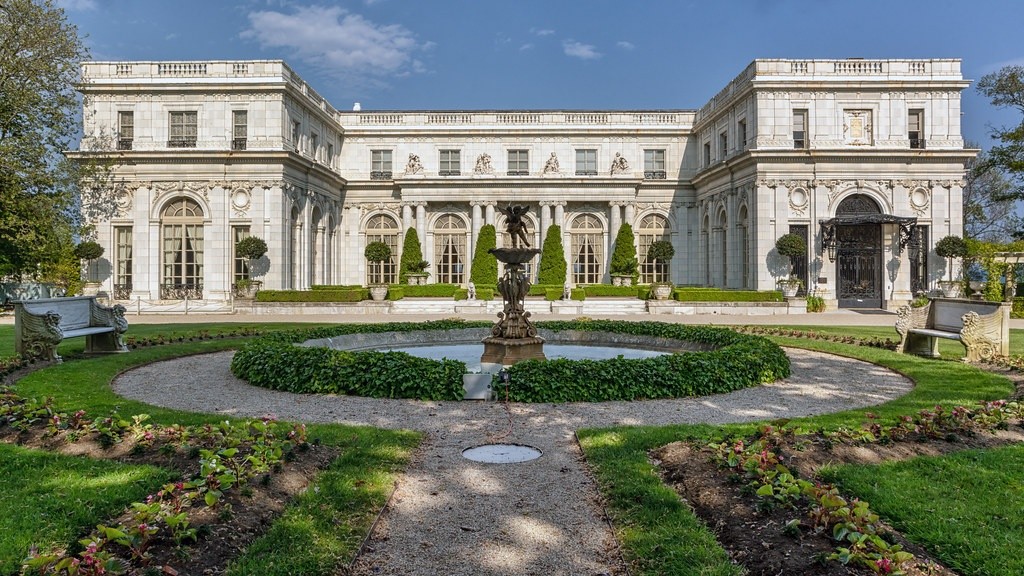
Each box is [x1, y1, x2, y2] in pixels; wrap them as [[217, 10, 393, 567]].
[[907, 240, 919, 263], [827, 238, 839, 263]]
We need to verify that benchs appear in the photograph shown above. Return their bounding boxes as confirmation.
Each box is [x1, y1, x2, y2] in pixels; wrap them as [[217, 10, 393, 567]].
[[13, 296, 129, 367], [896, 296, 1012, 364]]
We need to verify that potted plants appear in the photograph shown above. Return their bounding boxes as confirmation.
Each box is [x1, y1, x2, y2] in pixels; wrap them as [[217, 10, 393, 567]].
[[775, 234, 808, 297], [364, 241, 392, 302], [236, 236, 268, 299], [969, 281, 986, 296], [648, 241, 675, 300], [404, 260, 432, 286], [610, 262, 635, 287], [934, 235, 968, 297], [74, 241, 105, 297]]
